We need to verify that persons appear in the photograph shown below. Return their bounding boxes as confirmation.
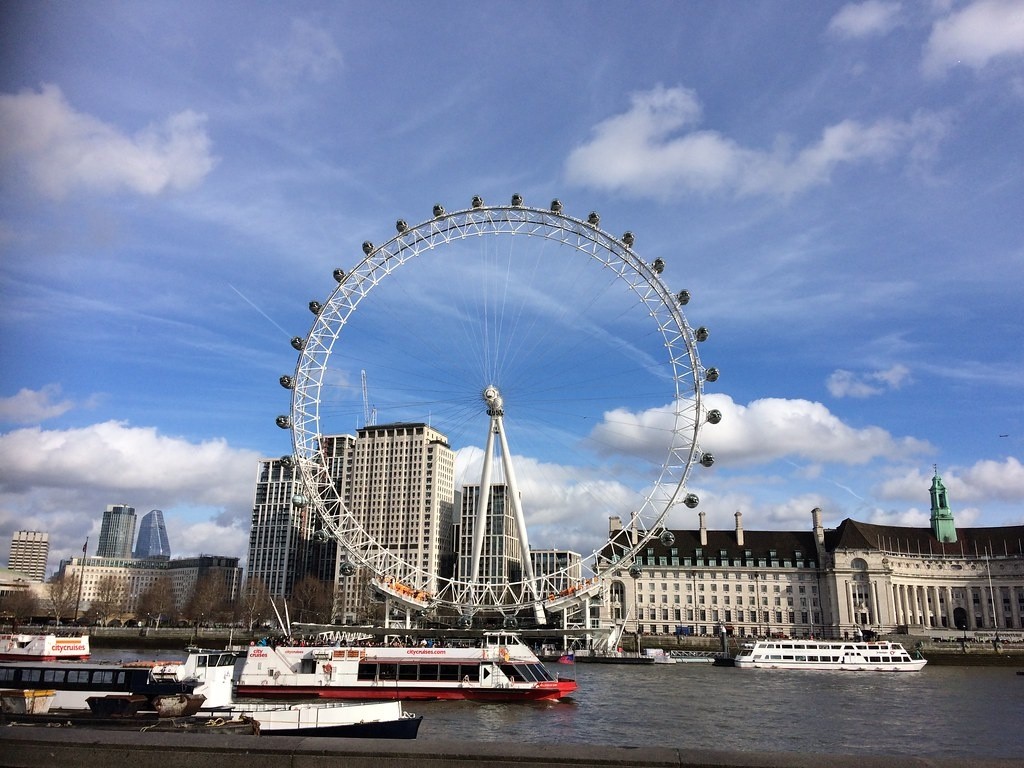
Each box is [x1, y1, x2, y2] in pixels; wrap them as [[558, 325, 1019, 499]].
[[444, 642, 469, 649], [258, 634, 332, 647], [332, 637, 436, 648], [499, 645, 510, 661]]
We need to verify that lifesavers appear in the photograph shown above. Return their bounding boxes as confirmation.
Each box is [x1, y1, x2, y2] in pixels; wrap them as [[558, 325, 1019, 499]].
[[324, 664, 332, 673], [889, 651, 894, 655]]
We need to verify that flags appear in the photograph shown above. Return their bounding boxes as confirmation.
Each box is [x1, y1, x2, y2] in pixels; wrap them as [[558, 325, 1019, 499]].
[[82, 541, 88, 552]]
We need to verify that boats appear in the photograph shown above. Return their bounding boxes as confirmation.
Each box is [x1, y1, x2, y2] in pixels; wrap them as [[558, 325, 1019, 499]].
[[0, 631, 423, 739], [221, 631, 579, 700], [733, 640, 928, 672]]
